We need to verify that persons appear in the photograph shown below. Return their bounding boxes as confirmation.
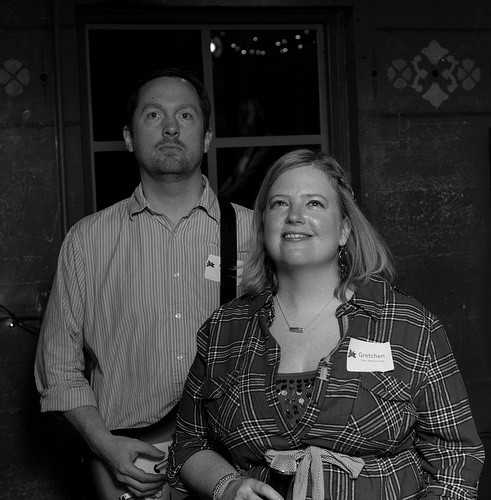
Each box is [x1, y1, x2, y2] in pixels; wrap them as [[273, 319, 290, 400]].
[[36, 72, 269, 500], [163, 148, 488, 500]]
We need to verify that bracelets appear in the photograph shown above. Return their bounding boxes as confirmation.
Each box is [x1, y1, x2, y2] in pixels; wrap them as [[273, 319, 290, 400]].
[[212, 471, 242, 500]]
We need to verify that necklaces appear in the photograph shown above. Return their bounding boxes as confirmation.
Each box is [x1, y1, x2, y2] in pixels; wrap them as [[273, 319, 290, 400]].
[[275, 268, 344, 333]]
